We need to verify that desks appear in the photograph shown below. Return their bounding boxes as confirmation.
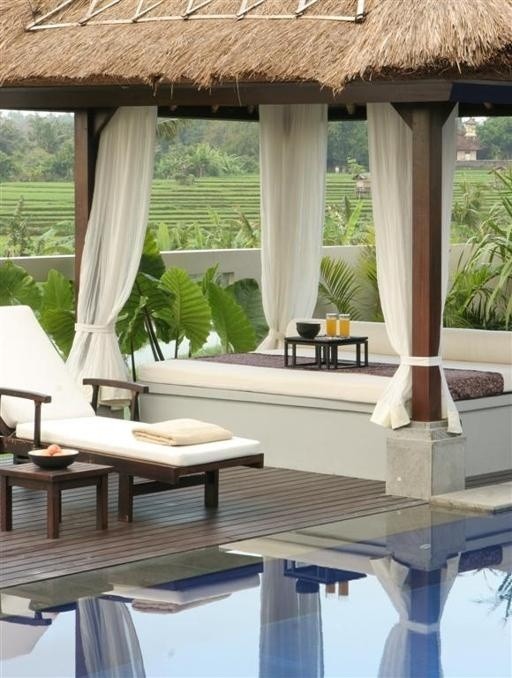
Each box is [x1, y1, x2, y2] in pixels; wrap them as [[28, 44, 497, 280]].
[[0, 458, 116, 539], [283, 564, 366, 585], [284, 334, 368, 371]]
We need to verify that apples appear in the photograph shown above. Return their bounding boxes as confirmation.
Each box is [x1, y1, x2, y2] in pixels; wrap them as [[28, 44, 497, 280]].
[[38, 444, 65, 457]]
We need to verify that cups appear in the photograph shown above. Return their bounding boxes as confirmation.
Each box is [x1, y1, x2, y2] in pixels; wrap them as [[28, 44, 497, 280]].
[[326, 313, 336, 337], [339, 313, 351, 338]]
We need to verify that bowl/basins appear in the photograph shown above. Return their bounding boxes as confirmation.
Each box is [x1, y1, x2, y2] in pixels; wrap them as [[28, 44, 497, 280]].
[[296, 322, 322, 340], [26, 448, 83, 471]]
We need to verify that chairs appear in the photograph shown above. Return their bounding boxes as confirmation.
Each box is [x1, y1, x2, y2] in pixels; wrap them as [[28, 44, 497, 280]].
[[0, 573, 260, 626], [0, 305, 272, 521]]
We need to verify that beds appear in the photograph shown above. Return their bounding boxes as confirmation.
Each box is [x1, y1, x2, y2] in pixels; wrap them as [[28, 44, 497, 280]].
[[124, 317, 512, 498]]
